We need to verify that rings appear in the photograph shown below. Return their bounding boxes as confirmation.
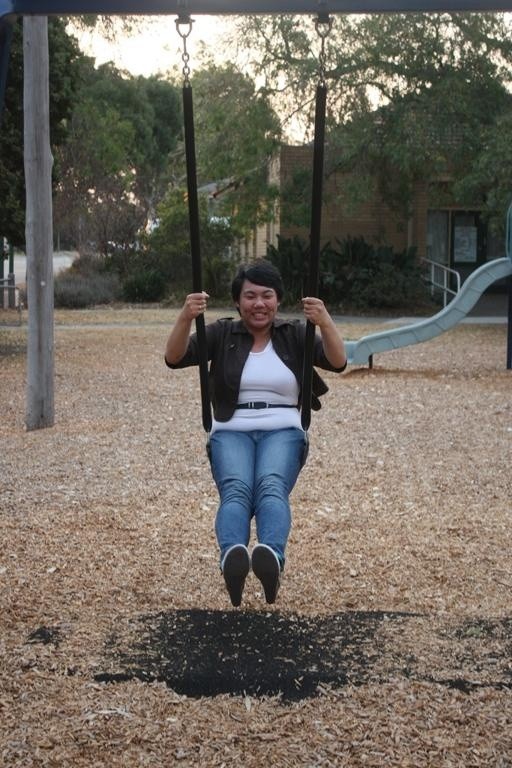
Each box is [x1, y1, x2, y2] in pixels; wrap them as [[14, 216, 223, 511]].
[[198, 305, 202, 311]]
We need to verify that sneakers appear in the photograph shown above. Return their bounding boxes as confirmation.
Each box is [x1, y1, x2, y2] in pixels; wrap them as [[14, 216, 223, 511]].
[[222, 543, 251, 606], [252, 544, 281, 604]]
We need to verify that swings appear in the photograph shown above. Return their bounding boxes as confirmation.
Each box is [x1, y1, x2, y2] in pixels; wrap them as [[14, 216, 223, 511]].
[[174, 15, 335, 467]]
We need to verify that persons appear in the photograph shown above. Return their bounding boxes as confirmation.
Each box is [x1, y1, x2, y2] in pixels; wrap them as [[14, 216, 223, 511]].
[[165, 260, 346, 606]]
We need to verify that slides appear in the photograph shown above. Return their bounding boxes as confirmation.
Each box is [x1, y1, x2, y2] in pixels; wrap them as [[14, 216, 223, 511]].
[[343, 257, 512, 364]]
